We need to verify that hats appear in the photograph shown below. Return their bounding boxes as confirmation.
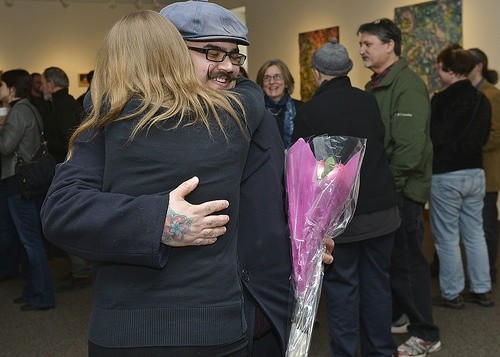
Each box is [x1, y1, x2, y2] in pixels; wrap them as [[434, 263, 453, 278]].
[[313, 38, 353, 76], [159, 1, 251, 46]]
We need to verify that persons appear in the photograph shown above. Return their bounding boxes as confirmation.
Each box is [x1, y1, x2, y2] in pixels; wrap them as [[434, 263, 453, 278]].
[[39, 0, 335, 357], [83, 10, 265, 357], [429, 43, 493, 309], [0, 69, 57, 310], [0, 67, 94, 282], [429, 48, 500, 288], [357, 18, 441, 357], [256, 59, 320, 328], [290, 37, 402, 357]]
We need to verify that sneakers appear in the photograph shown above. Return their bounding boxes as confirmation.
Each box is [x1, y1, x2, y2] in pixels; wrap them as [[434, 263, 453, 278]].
[[391, 314, 410, 333], [396, 335, 442, 357], [432, 290, 465, 309], [464, 292, 495, 307]]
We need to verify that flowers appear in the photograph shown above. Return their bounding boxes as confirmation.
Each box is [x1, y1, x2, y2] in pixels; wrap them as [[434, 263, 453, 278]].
[[282, 136, 367, 357]]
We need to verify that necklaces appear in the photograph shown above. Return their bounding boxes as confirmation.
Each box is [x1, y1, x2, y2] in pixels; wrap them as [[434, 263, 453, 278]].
[[266, 106, 283, 116]]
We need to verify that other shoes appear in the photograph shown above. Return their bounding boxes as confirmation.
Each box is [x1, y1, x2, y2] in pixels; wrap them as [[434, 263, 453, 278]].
[[54, 277, 91, 291], [21, 303, 43, 311], [0, 275, 14, 281], [14, 297, 29, 304]]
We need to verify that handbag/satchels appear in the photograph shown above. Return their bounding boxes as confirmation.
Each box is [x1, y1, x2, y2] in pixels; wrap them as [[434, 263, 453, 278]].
[[11, 103, 56, 198]]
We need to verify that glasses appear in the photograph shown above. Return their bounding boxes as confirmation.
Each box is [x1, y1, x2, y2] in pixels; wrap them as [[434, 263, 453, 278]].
[[371, 19, 392, 32], [262, 75, 284, 81], [187, 46, 246, 66], [310, 66, 316, 72]]
